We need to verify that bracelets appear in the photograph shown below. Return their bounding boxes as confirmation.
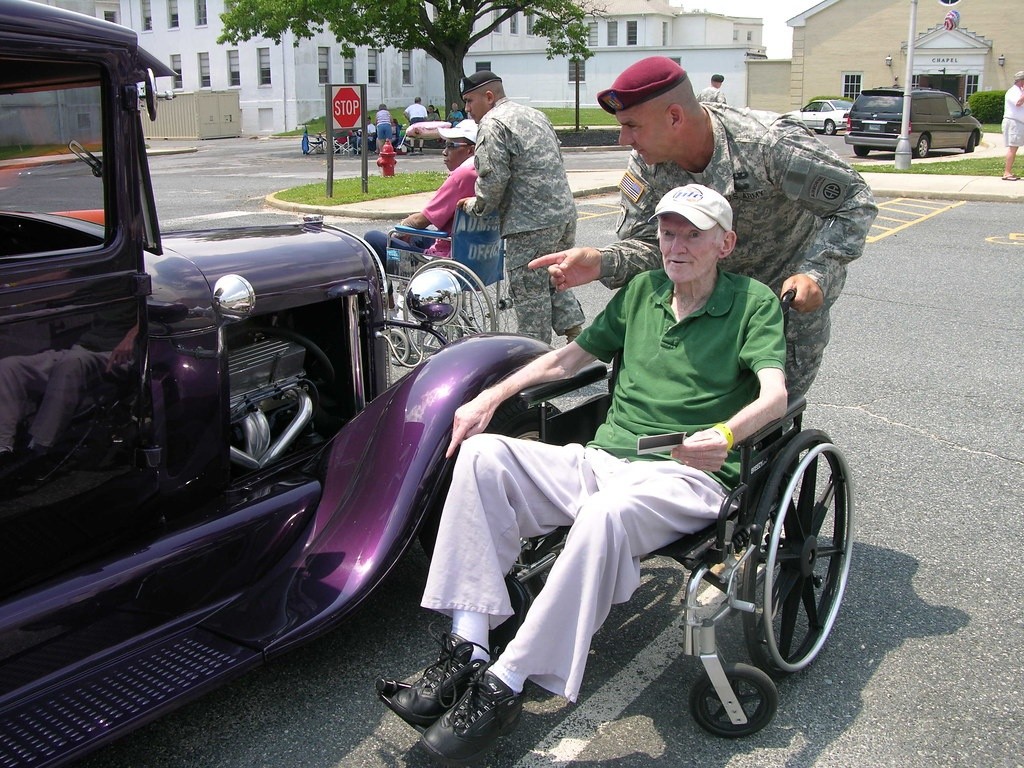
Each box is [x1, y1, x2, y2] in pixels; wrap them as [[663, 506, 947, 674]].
[[713, 424, 734, 452]]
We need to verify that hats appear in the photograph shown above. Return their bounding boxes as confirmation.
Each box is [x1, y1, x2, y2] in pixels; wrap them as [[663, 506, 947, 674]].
[[1014, 71, 1024, 80], [711, 74, 724, 82], [459, 70, 502, 95], [437, 118, 478, 144], [647, 184, 734, 232], [599, 57, 686, 116]]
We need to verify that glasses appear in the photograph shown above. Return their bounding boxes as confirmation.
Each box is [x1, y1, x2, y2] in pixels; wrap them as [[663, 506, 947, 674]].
[[443, 141, 467, 149]]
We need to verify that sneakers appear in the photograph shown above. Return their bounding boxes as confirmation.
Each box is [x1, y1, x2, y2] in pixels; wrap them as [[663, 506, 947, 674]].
[[418, 660, 525, 766], [390, 631, 489, 725]]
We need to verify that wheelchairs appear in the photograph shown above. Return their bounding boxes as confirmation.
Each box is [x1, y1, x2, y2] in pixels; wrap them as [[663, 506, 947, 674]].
[[376, 289, 855, 739], [383, 203, 521, 365]]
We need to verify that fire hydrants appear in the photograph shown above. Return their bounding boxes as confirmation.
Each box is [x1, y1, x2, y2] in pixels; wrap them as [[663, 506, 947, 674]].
[[377, 137, 397, 176]]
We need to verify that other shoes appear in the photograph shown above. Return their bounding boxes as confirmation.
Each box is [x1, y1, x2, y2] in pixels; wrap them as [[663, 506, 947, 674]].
[[419, 151, 423, 155], [410, 151, 415, 155]]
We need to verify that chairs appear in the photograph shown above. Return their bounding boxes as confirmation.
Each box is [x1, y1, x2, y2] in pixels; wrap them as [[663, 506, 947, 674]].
[[302, 123, 404, 156]]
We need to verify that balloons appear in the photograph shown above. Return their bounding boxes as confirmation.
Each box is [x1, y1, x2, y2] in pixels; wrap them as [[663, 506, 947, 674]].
[[944, 11, 960, 31]]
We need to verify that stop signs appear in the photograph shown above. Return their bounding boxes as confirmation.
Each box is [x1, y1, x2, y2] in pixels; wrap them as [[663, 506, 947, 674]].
[[333, 88, 361, 128]]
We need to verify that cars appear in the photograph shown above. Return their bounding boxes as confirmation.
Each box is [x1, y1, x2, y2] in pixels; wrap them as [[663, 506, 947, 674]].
[[845, 87, 982, 158], [0, 1, 554, 768], [783, 100, 854, 135]]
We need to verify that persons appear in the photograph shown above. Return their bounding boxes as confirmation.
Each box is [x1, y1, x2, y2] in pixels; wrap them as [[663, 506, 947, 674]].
[[456, 71, 585, 346], [0, 227, 140, 481], [1001, 71, 1024, 181], [697, 74, 726, 104], [334, 97, 464, 157], [527, 57, 878, 441], [393, 186, 788, 762], [363, 119, 478, 278]]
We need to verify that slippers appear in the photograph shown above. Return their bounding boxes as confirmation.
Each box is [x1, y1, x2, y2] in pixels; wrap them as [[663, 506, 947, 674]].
[[1001, 174, 1021, 181]]
